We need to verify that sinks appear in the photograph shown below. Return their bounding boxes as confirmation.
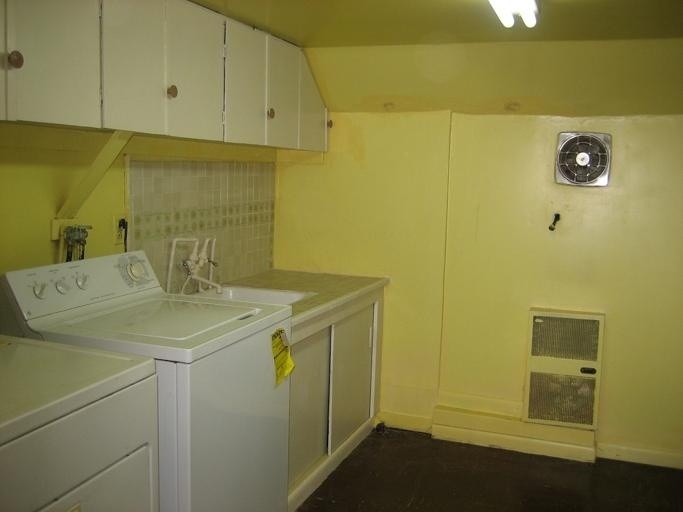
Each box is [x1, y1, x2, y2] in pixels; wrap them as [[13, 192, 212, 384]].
[[195, 286, 319, 304]]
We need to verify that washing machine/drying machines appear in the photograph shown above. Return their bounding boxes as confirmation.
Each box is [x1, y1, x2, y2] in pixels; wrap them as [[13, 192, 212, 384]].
[[0, 248, 292, 512]]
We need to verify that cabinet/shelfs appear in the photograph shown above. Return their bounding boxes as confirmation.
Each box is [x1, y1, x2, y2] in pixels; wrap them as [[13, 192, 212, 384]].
[[301, 51, 331, 152], [287, 303, 377, 503], [0, 375, 155, 512], [226, 16, 300, 151], [0, 0, 101, 131], [101, 0, 226, 143]]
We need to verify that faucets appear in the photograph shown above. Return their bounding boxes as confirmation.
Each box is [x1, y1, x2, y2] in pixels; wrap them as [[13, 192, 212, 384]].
[[192, 275, 222, 294]]
